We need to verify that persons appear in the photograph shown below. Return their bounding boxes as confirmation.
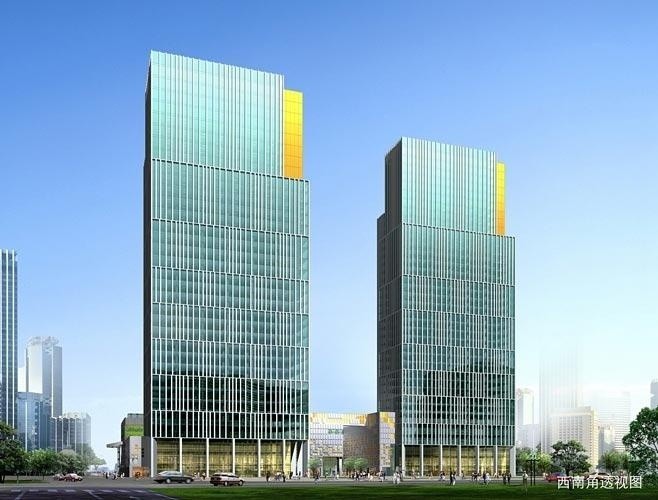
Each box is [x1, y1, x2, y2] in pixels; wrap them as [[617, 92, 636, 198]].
[[353, 468, 403, 486], [266, 471, 293, 483], [522, 471, 529, 486], [507, 470, 512, 484], [502, 471, 507, 484], [194, 471, 205, 480], [105, 472, 125, 480], [441, 467, 499, 485]]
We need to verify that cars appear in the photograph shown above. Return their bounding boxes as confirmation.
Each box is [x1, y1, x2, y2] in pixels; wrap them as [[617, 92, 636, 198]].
[[53, 472, 84, 482], [153, 470, 194, 483], [545, 471, 615, 481]]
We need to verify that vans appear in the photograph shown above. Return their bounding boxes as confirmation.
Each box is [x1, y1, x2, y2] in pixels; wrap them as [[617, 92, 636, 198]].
[[210, 473, 246, 487]]
